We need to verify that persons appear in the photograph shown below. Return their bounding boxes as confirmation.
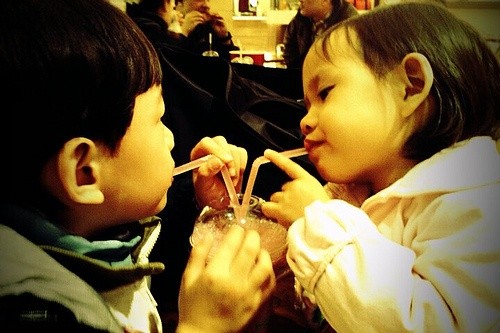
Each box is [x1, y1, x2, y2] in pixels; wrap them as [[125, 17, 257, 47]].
[[0, 0, 500, 333]]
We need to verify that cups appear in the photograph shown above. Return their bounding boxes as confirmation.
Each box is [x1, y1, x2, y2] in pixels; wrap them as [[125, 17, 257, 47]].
[[190, 194, 290, 280]]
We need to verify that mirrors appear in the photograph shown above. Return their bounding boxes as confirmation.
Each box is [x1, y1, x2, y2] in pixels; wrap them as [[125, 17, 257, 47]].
[[233, 0, 262, 20]]
